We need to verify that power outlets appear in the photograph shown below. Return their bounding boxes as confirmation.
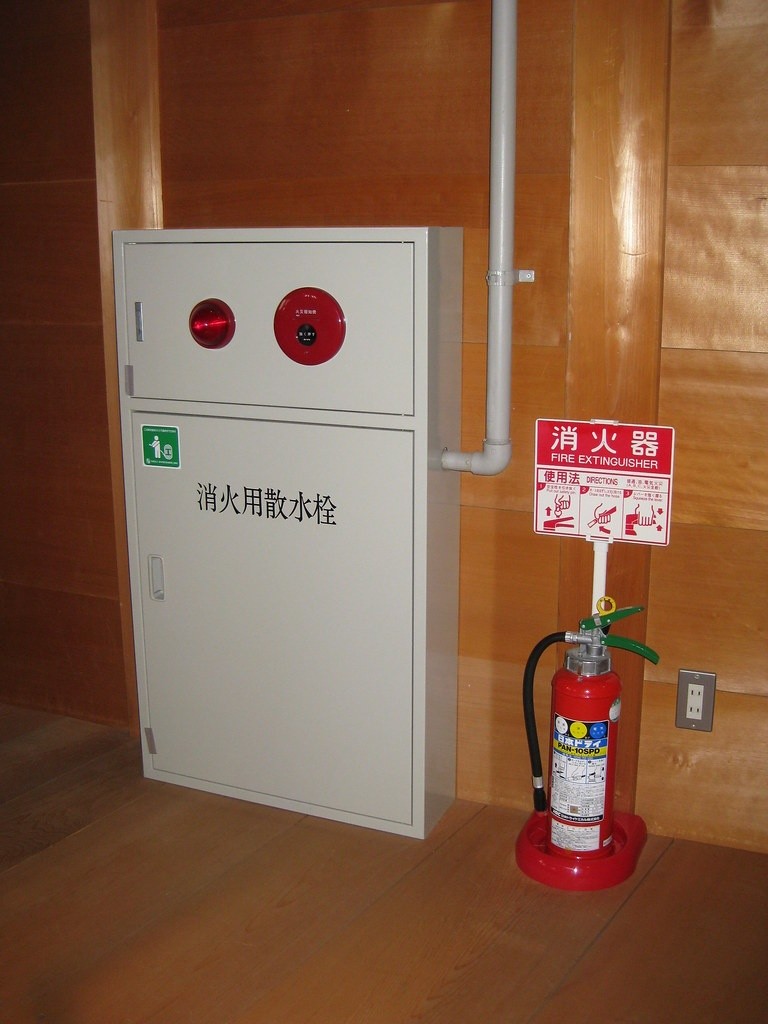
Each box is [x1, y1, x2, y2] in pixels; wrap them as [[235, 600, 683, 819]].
[[674, 668, 716, 732]]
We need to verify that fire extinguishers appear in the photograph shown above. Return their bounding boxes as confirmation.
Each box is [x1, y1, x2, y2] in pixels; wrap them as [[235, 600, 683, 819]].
[[521, 596, 659, 863]]
